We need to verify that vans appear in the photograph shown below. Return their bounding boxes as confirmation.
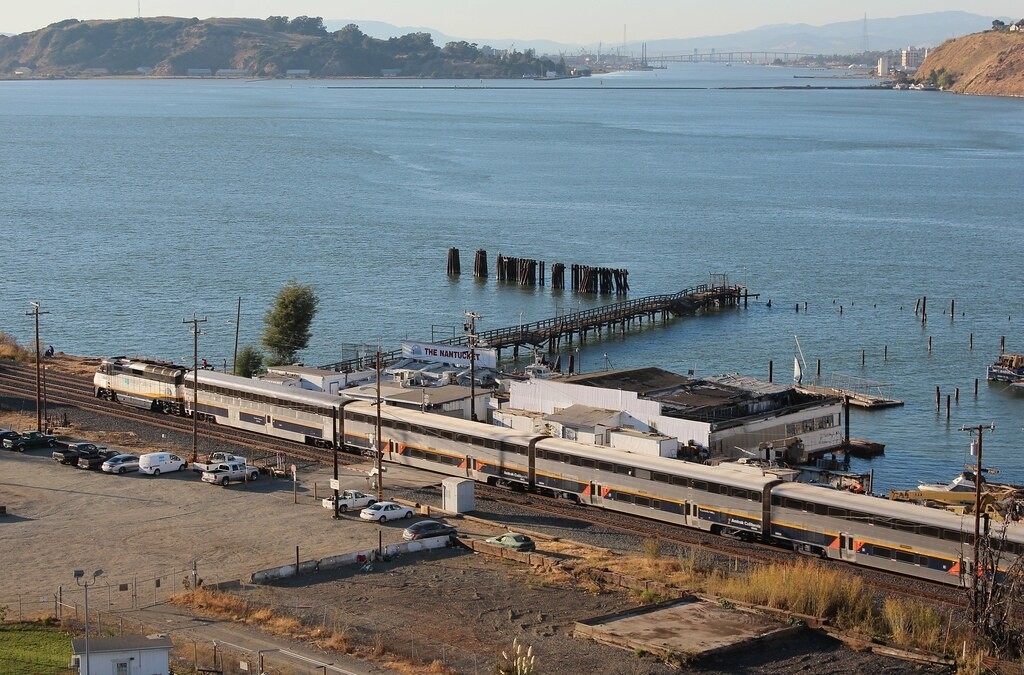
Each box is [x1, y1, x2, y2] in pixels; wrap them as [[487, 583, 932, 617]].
[[139, 451, 188, 475]]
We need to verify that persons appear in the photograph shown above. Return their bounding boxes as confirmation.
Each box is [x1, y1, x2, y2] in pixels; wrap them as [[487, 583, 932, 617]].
[[49, 345, 54, 355], [202, 359, 207, 368], [224, 358, 226, 365]]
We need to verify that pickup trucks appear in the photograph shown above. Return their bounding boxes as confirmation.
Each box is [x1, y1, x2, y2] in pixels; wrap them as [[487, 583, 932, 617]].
[[3, 429, 58, 452], [77, 450, 121, 470], [322, 489, 378, 514], [52, 443, 107, 465], [192, 451, 246, 474], [200, 463, 260, 486]]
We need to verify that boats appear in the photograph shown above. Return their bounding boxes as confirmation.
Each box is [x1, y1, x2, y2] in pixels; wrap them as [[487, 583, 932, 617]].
[[986, 353, 1024, 387], [889, 463, 1024, 506], [523, 347, 564, 380]]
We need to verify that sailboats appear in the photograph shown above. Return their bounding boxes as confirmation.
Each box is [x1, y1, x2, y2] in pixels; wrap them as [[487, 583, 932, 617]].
[[791, 334, 808, 393]]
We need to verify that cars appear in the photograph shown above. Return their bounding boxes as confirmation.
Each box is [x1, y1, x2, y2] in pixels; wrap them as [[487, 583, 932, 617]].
[[402, 519, 457, 541], [360, 500, 415, 524], [486, 531, 536, 554], [0, 427, 19, 448], [102, 453, 139, 474]]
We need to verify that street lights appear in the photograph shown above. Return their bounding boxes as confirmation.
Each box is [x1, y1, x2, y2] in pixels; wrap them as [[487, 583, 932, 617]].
[[73, 569, 103, 673], [226, 320, 241, 374], [181, 355, 199, 463], [971, 468, 1004, 631], [358, 385, 382, 502]]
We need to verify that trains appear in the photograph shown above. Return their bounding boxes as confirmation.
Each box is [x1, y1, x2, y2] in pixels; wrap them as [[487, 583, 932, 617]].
[[92, 355, 1024, 601]]
